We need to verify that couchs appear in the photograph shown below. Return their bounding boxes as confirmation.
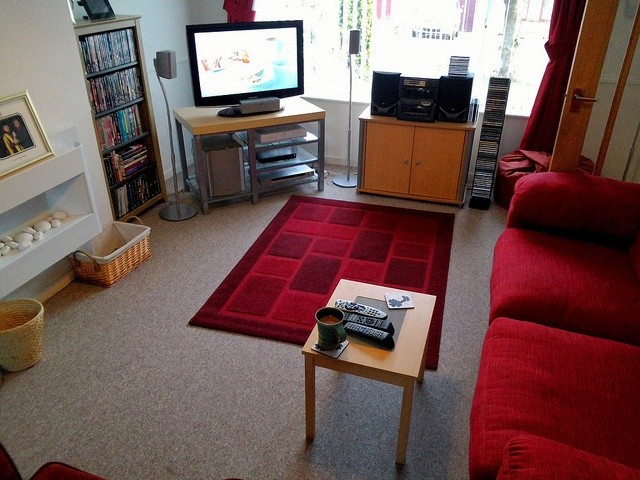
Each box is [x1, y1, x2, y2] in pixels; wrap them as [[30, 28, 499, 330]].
[[0, 444, 104, 478], [469, 172, 640, 480]]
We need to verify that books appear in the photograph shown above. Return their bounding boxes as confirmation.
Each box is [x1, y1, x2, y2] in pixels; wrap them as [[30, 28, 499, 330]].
[[96, 104, 143, 151], [448, 56, 470, 78], [78, 29, 138, 73], [103, 143, 149, 186], [87, 66, 143, 114], [110, 168, 161, 217]]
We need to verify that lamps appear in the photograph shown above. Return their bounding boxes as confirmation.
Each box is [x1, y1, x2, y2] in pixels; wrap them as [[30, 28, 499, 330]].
[[332, 29, 360, 187], [154, 50, 198, 223]]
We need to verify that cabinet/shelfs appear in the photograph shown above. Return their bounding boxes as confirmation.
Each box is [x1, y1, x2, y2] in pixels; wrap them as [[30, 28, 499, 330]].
[[0, 141, 103, 301], [173, 99, 327, 215], [74, 13, 167, 223], [356, 105, 477, 207]]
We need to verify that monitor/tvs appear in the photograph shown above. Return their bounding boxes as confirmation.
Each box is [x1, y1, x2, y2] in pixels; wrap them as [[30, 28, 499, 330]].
[[185, 20, 304, 118]]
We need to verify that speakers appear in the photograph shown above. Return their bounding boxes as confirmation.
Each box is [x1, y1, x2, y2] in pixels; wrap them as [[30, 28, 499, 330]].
[[437, 76, 473, 123], [156, 50, 177, 79], [349, 30, 359, 54], [371, 71, 402, 117]]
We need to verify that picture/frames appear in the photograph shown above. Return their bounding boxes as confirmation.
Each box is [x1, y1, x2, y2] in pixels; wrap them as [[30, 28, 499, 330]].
[[82, 0, 116, 22], [0, 90, 56, 178]]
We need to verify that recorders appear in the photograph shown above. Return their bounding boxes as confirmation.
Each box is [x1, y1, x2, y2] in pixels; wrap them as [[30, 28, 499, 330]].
[[397, 76, 439, 123]]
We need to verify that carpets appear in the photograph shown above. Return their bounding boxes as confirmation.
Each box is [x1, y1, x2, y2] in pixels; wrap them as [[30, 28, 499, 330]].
[[185, 192, 455, 372]]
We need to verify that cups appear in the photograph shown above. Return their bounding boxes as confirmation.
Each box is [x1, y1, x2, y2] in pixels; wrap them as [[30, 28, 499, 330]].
[[314, 307, 345, 351]]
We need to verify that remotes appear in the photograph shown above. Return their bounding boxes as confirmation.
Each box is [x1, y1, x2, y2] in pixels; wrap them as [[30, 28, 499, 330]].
[[343, 312, 392, 332], [344, 322, 390, 342], [335, 299, 388, 320]]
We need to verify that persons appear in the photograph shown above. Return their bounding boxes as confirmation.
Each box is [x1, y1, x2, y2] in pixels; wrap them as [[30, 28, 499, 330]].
[[2, 123, 25, 156], [13, 118, 34, 148]]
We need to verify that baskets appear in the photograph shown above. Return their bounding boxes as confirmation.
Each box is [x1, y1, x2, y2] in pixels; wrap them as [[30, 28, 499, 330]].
[[0, 298, 44, 372]]
